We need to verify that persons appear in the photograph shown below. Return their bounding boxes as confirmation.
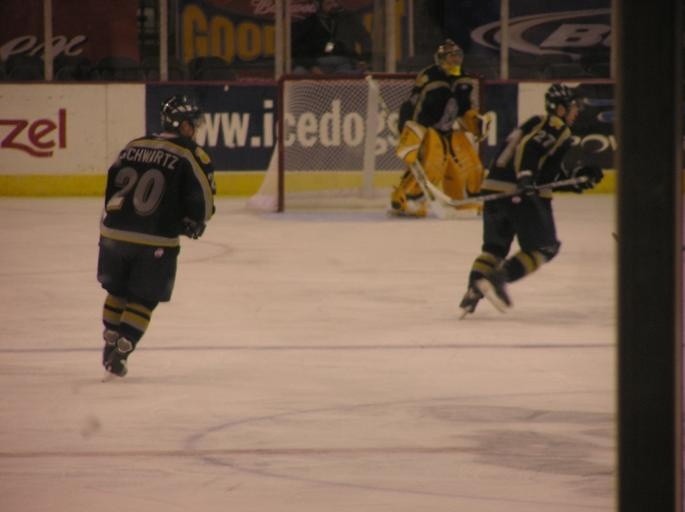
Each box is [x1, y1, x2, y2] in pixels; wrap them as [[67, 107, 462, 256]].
[[95, 90, 217, 378], [390, 36, 505, 220], [290, 1, 373, 76], [457, 78, 618, 316]]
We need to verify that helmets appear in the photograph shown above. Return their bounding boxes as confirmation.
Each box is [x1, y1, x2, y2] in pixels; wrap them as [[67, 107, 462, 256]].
[[544, 84, 582, 114], [159, 94, 204, 132], [434, 40, 463, 60]]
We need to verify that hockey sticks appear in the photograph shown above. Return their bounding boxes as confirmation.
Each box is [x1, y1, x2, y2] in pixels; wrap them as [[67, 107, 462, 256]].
[[366, 75, 484, 220], [427, 173, 590, 207]]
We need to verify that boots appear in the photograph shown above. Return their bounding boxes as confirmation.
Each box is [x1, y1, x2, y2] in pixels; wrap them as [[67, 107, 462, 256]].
[[474, 276, 514, 313], [460, 286, 480, 313], [103, 348, 127, 377]]
